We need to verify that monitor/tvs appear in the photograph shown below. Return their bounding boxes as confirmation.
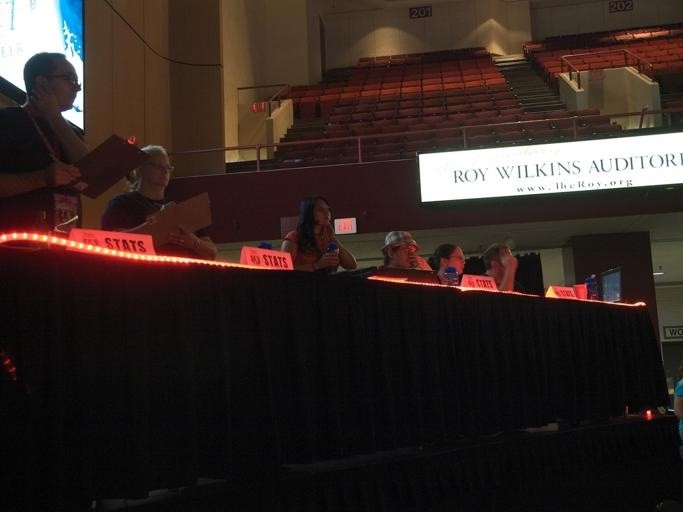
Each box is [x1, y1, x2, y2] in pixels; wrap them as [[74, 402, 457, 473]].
[[599, 265, 623, 303], [334, 217, 357, 235]]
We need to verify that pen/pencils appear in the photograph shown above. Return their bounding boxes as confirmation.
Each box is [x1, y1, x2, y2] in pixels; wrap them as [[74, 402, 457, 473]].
[[48, 153, 62, 164], [153, 202, 165, 209]]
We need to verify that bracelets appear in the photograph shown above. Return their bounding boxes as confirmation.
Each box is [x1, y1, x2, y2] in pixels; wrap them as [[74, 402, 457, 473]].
[[311, 260, 320, 271], [191, 236, 199, 253]]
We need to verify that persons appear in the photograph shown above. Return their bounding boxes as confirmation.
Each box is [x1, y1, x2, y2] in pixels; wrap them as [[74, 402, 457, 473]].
[[673, 364, 682, 461], [482, 244, 524, 291], [380, 231, 432, 270], [0, 51, 90, 241], [101, 143, 218, 262], [426, 243, 466, 286], [281, 195, 357, 273]]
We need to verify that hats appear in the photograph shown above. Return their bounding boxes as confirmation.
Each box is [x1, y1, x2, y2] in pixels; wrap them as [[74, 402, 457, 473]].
[[379, 230, 421, 259]]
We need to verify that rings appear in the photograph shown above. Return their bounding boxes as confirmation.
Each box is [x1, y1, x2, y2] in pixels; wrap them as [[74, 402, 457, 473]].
[[178, 238, 185, 244]]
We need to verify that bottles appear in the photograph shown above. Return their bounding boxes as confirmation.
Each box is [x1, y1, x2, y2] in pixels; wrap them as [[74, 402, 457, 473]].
[[325, 241, 339, 275], [585, 273, 601, 300], [442, 267, 460, 287]]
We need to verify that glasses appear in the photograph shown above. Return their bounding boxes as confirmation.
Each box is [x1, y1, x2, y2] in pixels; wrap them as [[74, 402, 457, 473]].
[[42, 74, 81, 86], [141, 162, 175, 172]]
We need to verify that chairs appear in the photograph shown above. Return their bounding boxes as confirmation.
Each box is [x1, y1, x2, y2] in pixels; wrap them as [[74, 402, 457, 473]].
[[518, 25, 681, 126], [270, 46, 623, 166]]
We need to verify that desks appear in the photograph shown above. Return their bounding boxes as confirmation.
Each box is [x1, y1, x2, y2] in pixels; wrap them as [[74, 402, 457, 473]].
[[0, 246, 658, 502]]
